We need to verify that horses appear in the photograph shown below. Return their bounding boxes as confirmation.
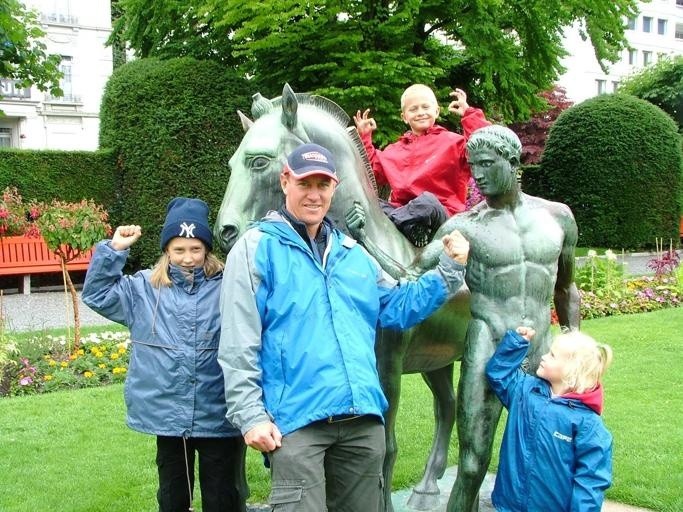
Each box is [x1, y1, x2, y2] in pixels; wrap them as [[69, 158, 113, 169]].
[[211, 81, 473, 511]]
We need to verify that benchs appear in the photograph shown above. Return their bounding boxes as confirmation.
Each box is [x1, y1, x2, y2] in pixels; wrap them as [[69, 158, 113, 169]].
[[0, 234, 95, 295]]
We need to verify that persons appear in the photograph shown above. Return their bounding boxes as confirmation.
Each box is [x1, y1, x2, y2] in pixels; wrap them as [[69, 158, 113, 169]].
[[484, 324, 611, 512], [80, 196, 251, 511], [216, 143, 471, 512], [351, 82, 496, 247], [345, 123, 583, 512]]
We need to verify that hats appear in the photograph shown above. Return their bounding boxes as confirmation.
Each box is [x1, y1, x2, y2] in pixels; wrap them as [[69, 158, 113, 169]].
[[160, 197, 213, 252], [282, 143, 340, 191]]
